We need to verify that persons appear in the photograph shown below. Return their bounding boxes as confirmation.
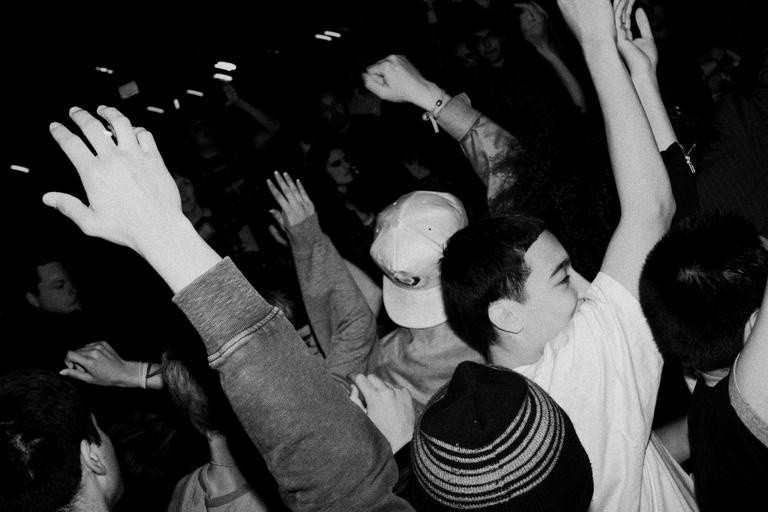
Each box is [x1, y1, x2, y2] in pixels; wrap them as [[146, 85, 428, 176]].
[[1, 1, 766, 512]]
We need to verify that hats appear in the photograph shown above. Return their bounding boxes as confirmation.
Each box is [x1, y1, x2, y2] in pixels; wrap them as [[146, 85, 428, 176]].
[[369, 190, 469, 329], [411, 360, 594, 510]]
